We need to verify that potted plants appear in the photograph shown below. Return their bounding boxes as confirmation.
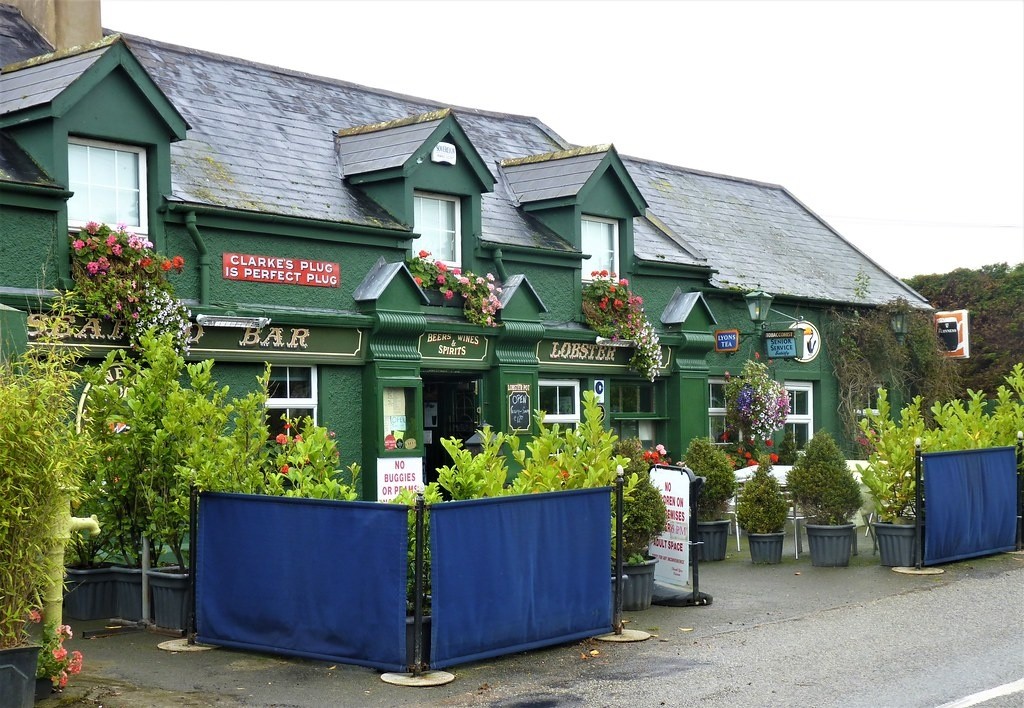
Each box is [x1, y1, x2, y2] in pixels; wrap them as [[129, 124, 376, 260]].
[[857, 387, 930, 566], [786, 427, 864, 568], [389, 483, 443, 670], [62, 326, 272, 630], [0, 362, 94, 708], [737, 455, 791, 564], [534, 391, 668, 618], [683, 436, 736, 562]]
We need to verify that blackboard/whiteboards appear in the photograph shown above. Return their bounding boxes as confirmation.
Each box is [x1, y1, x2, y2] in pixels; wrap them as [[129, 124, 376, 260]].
[[508, 391, 531, 431]]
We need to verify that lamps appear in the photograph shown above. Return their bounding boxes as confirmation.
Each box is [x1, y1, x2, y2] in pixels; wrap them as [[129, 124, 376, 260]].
[[887, 305, 910, 346], [742, 282, 775, 338]]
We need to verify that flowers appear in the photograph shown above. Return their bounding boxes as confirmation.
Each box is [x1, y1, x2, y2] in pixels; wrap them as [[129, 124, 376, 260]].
[[722, 353, 791, 441], [643, 443, 672, 466], [409, 250, 502, 328], [277, 424, 340, 475], [73, 221, 190, 357], [524, 458, 588, 493], [22, 609, 83, 687], [721, 432, 779, 468], [583, 270, 663, 383]]
